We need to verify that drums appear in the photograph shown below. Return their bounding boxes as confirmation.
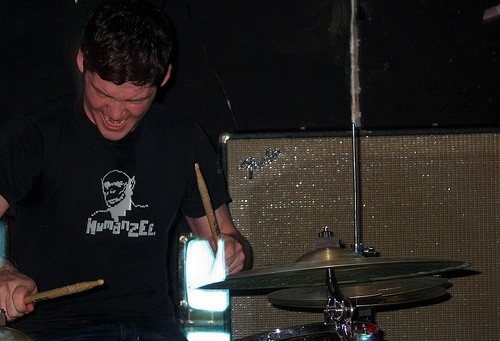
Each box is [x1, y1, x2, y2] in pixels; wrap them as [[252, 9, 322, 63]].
[[234, 320, 381, 341]]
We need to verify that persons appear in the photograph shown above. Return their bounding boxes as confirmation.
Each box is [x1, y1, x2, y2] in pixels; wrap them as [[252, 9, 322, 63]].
[[1, 0, 244, 340]]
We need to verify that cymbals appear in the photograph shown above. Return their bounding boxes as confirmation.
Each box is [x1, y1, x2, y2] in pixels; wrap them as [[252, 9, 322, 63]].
[[268, 276, 453, 307], [226, 245, 444, 279], [196, 260, 471, 288]]
